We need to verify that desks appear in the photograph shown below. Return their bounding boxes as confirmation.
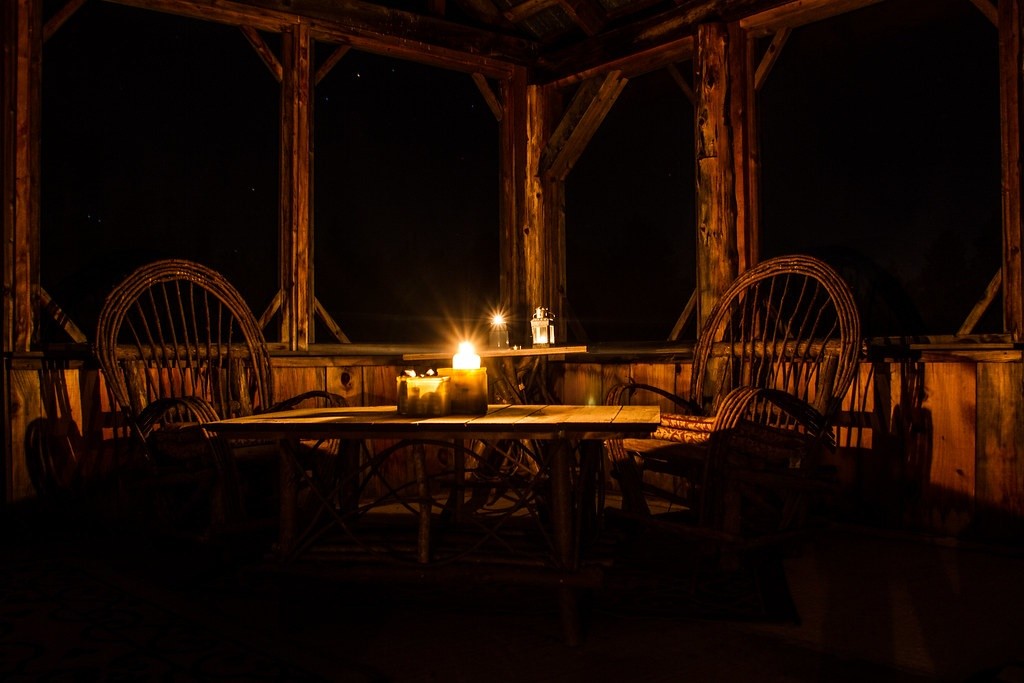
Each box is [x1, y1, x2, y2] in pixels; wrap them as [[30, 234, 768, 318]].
[[402, 345, 587, 508], [199, 405, 662, 646]]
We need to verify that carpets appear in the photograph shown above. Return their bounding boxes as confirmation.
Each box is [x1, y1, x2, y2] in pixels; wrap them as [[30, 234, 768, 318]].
[[594, 509, 802, 628]]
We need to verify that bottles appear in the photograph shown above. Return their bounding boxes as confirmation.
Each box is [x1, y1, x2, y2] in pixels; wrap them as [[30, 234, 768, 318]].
[[530, 306, 556, 348]]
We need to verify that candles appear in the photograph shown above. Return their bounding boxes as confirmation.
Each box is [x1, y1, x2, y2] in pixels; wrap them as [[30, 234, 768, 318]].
[[452, 338, 481, 371], [487, 311, 508, 348]]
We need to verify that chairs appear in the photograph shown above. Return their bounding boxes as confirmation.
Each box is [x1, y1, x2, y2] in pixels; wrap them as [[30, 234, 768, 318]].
[[94, 260, 353, 552], [601, 253, 861, 575]]
